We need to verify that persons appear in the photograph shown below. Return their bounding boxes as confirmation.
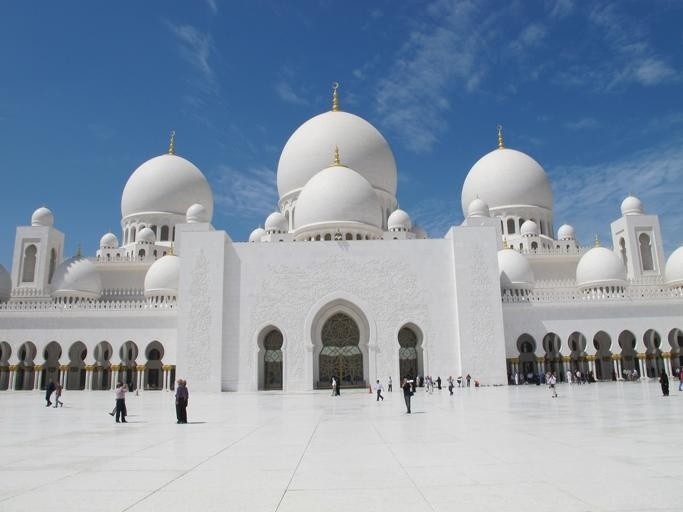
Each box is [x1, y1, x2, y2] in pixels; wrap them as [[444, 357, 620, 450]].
[[331, 377, 340, 396], [515, 372, 554, 385], [417, 375, 441, 393], [457, 374, 479, 387], [401, 377, 413, 413], [659, 369, 669, 396], [567, 369, 590, 385], [547, 375, 557, 398], [611, 369, 616, 381], [52, 380, 63, 408], [623, 368, 638, 381], [114, 383, 126, 423], [448, 376, 454, 395], [676, 369, 682, 391], [182, 380, 188, 422], [174, 378, 185, 423], [45, 378, 54, 406], [107, 381, 127, 416], [376, 380, 384, 400], [388, 377, 392, 392]]
